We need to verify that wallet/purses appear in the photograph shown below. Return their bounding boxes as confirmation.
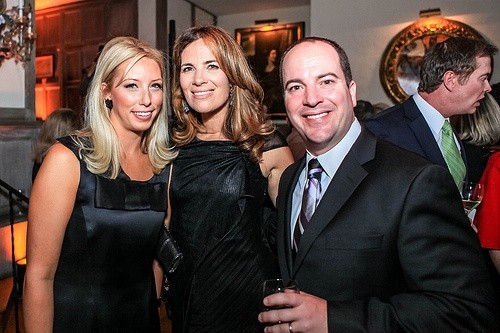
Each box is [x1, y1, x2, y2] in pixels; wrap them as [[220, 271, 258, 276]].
[[156, 226, 184, 275]]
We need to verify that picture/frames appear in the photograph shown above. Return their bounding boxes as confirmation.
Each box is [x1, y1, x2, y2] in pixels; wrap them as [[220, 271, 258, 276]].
[[235, 21, 306, 120], [379, 18, 494, 108]]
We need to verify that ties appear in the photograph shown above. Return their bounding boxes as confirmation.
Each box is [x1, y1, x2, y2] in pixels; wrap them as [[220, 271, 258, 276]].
[[441, 120, 466, 190], [291, 157, 324, 269]]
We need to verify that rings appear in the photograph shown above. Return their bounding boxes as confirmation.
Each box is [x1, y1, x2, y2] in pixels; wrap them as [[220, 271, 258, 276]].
[[289, 322, 294, 333]]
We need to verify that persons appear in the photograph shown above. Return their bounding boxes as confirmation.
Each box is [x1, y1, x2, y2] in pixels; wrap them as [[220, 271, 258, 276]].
[[286, 81, 500, 163], [361, 37, 500, 232], [258, 37, 500, 333], [22, 36, 179, 332], [259, 47, 284, 113], [449, 92, 500, 274], [152, 26, 295, 333], [32, 108, 84, 186]]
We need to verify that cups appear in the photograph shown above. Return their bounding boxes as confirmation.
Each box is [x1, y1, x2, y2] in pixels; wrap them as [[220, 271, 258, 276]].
[[457, 181, 484, 216], [263, 279, 300, 327]]
